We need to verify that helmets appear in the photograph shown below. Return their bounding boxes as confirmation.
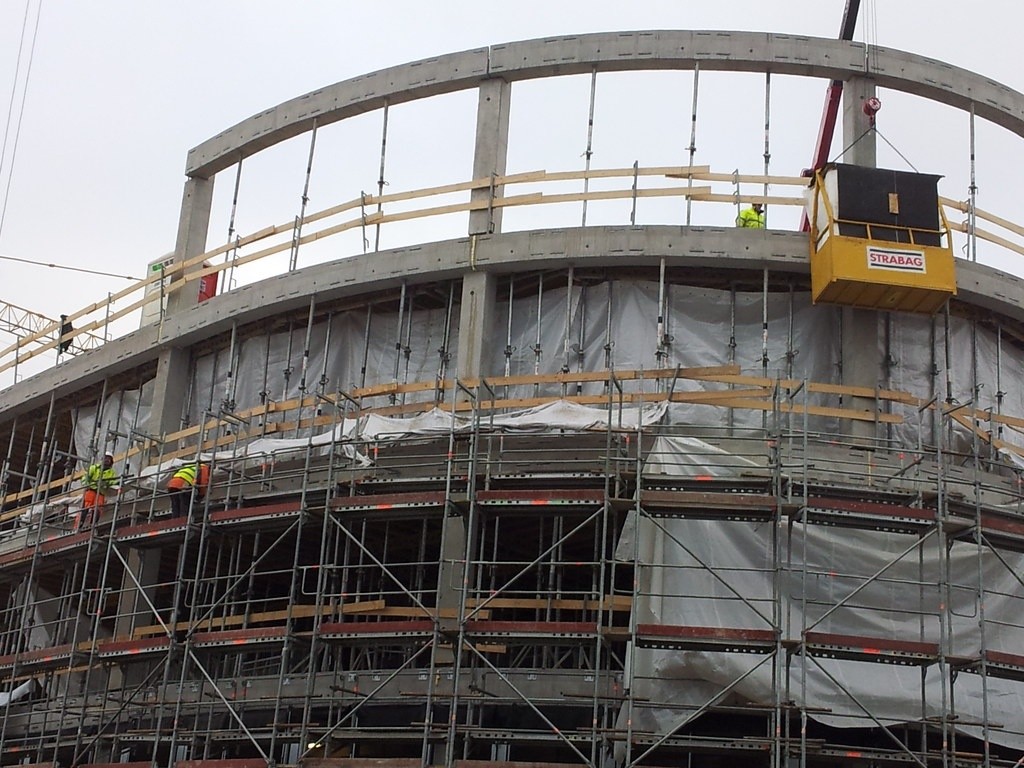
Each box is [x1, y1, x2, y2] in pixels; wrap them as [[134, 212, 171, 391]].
[[105, 451, 114, 458]]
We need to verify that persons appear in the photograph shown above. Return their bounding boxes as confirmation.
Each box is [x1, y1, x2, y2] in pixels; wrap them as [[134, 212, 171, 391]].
[[737, 204, 764, 229], [78, 451, 120, 529], [166, 463, 209, 517]]
